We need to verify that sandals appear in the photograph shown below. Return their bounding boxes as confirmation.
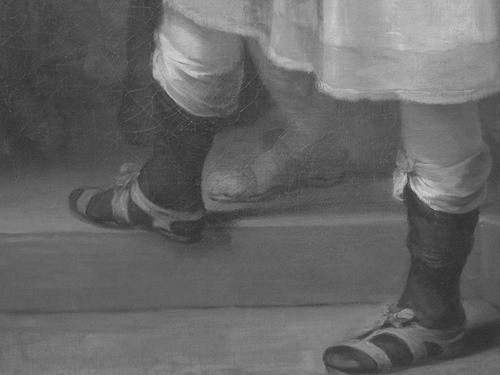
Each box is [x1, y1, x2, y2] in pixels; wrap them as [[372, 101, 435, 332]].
[[324, 306, 471, 375], [66, 168, 209, 244], [207, 137, 351, 202]]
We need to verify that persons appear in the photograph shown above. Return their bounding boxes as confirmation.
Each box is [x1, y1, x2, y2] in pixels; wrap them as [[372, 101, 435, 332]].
[[67, 0, 499, 374]]
